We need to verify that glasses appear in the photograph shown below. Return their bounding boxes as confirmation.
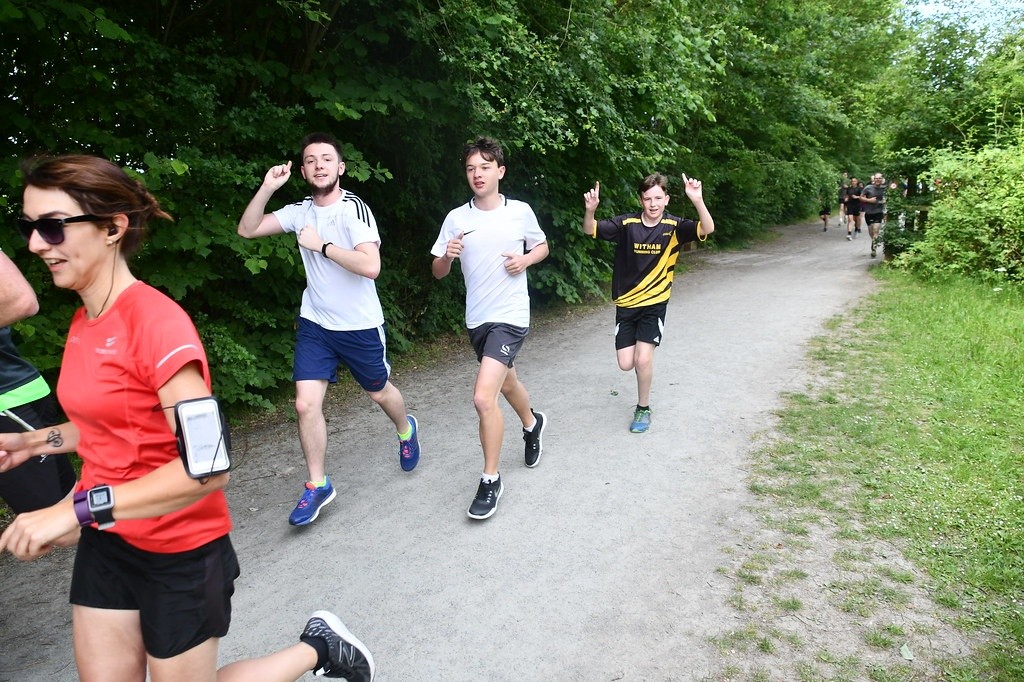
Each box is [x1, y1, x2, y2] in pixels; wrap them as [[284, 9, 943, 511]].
[[16, 212, 101, 245]]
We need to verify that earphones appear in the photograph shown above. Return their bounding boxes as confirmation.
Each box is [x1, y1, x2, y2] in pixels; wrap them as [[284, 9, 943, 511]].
[[107, 224, 117, 237]]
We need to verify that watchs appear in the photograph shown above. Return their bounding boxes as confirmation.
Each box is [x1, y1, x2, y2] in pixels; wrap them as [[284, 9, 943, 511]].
[[87, 483, 116, 530]]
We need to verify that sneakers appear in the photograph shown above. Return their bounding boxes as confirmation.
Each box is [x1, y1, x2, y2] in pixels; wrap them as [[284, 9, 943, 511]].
[[522, 408, 547, 467], [396, 414, 422, 473], [467, 471, 504, 520], [630, 405, 653, 434], [288, 475, 337, 526], [299, 610, 376, 682]]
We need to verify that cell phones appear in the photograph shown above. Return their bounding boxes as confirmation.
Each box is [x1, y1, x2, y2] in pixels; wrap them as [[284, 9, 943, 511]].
[[178, 399, 231, 477]]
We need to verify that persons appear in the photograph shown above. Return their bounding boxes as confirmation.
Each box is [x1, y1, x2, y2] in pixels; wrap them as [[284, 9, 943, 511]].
[[431, 137, 549, 519], [583, 172, 715, 434], [0, 249, 81, 545], [237, 133, 421, 525], [818, 173, 908, 258], [0, 155, 376, 682]]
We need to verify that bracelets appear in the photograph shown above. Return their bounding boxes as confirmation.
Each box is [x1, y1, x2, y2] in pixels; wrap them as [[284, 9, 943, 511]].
[[322, 242, 334, 258], [73, 490, 95, 527]]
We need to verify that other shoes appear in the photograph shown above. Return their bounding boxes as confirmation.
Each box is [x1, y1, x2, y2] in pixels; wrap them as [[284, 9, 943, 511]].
[[854, 234, 858, 239], [846, 234, 852, 241], [871, 250, 876, 257]]
